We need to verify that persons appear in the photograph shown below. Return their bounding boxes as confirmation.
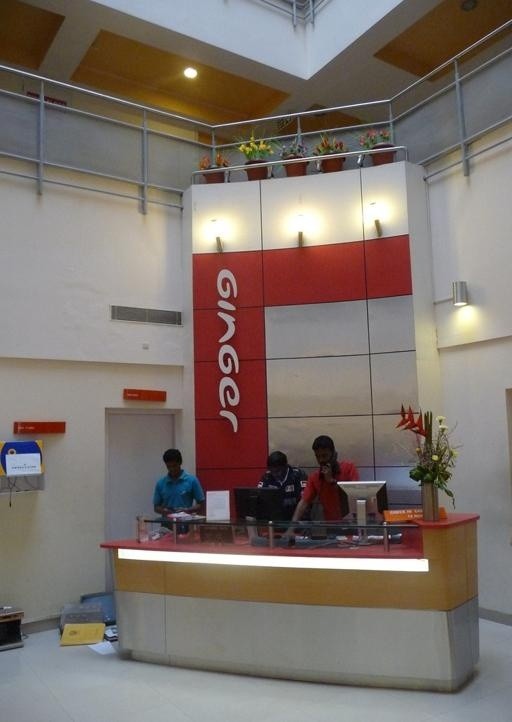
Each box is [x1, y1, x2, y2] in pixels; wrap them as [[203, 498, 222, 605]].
[[283, 436, 359, 538], [153, 449, 205, 535], [256, 452, 311, 518]]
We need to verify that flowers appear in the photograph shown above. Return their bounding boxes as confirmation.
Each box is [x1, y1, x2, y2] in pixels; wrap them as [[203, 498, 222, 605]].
[[198, 152, 226, 168], [315, 139, 347, 156], [280, 142, 309, 157], [237, 138, 273, 160], [357, 131, 390, 148], [396, 407, 454, 499]]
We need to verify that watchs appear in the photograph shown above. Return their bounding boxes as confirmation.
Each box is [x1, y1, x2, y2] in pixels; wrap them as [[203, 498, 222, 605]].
[[162, 509, 168, 514]]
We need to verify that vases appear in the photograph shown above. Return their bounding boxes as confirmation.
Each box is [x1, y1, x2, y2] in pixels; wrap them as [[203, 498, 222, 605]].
[[284, 156, 309, 177], [245, 160, 268, 180], [206, 165, 228, 183], [370, 144, 396, 165], [321, 154, 344, 173], [421, 481, 439, 524]]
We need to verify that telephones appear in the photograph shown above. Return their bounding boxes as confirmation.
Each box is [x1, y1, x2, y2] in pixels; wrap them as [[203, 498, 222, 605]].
[[319, 452, 338, 479]]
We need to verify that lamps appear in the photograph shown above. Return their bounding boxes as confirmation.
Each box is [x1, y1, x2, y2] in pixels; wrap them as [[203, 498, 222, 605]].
[[451, 281, 466, 306]]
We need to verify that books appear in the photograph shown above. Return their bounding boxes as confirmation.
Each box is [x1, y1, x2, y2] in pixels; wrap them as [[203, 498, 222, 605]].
[[60, 624, 105, 647]]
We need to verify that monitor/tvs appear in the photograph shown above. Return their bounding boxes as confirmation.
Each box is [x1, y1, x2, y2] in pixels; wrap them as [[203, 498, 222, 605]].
[[233, 487, 286, 544], [336, 480, 391, 547]]
